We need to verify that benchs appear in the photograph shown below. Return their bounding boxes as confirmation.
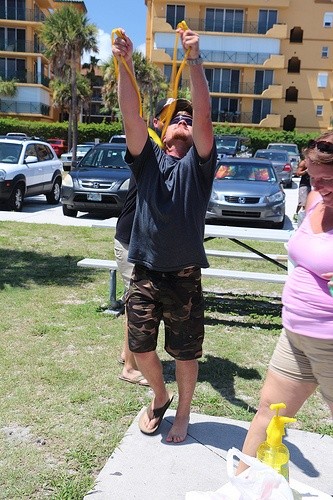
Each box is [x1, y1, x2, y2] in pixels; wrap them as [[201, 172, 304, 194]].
[[206, 250, 288, 261], [77, 258, 288, 309]]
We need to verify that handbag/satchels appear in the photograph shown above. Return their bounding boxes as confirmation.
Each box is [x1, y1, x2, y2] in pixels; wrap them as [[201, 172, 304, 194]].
[[185, 447, 294, 500]]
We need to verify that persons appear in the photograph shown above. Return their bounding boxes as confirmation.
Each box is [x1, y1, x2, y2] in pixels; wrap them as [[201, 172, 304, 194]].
[[236, 129, 333, 476], [111, 28, 218, 442], [113, 98, 176, 386], [293, 159, 311, 220]]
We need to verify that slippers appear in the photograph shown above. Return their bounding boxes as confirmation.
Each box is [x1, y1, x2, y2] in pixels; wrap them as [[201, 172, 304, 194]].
[[138, 389, 174, 434], [118, 358, 125, 364], [119, 373, 150, 386]]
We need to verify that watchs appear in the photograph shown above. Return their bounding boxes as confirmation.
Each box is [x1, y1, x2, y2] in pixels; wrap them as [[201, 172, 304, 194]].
[[186, 54, 204, 65]]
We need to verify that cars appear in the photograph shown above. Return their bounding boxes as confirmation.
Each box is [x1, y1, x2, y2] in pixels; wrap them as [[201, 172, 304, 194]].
[[0, 130, 127, 172], [205, 135, 302, 189], [204, 157, 287, 229]]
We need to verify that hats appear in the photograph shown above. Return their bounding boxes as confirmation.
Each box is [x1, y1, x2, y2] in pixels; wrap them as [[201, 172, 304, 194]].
[[154, 97, 177, 117], [161, 98, 193, 125]]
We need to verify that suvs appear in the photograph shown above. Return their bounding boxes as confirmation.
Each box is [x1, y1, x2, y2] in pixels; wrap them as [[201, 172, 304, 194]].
[[59, 141, 137, 219], [0, 137, 64, 213]]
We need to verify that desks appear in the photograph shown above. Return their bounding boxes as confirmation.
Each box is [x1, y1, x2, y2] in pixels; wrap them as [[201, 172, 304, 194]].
[[204, 225, 292, 241]]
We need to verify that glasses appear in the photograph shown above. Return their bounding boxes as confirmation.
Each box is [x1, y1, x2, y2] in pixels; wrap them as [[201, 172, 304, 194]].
[[170, 114, 193, 126], [307, 139, 333, 153]]
